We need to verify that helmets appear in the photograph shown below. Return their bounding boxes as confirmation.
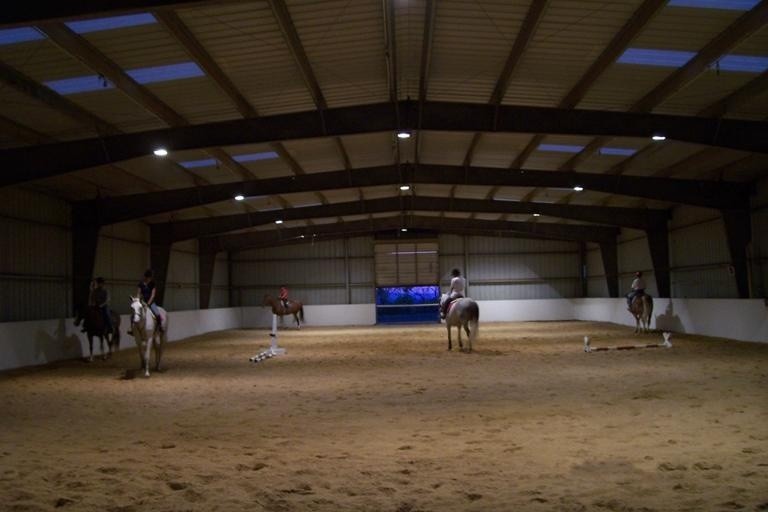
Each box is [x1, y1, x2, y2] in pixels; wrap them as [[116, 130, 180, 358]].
[[451, 268, 461, 276], [633, 272, 642, 277], [145, 270, 152, 278], [96, 277, 106, 283]]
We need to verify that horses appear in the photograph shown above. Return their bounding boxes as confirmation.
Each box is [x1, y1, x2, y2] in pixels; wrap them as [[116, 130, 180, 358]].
[[73, 303, 120, 361], [263, 293, 305, 329], [438, 294, 479, 353], [627, 293, 654, 335], [128, 294, 169, 377]]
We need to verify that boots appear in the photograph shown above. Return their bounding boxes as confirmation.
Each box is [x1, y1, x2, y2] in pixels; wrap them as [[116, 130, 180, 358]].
[[155, 314, 164, 332], [127, 330, 133, 336]]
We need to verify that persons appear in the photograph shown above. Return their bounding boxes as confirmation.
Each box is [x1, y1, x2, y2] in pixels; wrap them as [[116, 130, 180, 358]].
[[624, 271, 645, 312], [439, 267, 465, 319], [126, 266, 165, 338], [72, 276, 114, 333], [277, 284, 289, 314]]
